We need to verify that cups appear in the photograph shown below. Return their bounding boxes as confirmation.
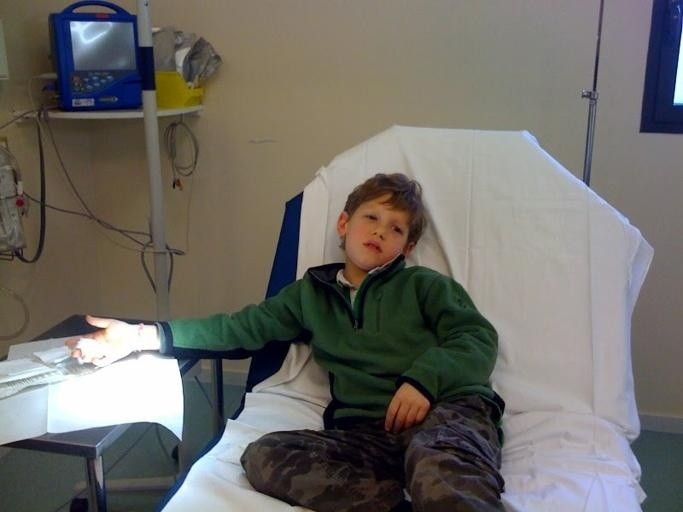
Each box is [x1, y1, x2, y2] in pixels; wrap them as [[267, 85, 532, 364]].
[[155, 70, 194, 109]]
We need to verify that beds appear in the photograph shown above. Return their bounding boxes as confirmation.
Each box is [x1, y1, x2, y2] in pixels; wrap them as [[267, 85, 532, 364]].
[[163, 119, 641, 512]]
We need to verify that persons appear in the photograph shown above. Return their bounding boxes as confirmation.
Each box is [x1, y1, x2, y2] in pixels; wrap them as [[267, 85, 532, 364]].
[[63, 172, 508, 512]]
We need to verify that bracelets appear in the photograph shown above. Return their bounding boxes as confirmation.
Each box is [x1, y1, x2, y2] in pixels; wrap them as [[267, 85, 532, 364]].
[[134, 323, 144, 352]]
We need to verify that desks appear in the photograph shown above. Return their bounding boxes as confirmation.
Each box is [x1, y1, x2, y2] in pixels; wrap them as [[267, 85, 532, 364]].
[[0, 313, 229, 510]]
